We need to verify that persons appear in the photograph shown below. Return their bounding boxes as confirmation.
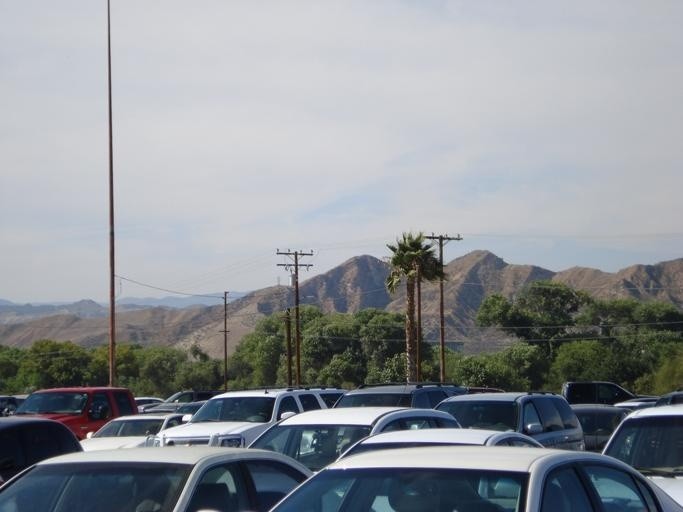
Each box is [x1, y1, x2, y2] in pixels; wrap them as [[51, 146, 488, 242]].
[[24, 383, 37, 394], [2, 400, 17, 417]]
